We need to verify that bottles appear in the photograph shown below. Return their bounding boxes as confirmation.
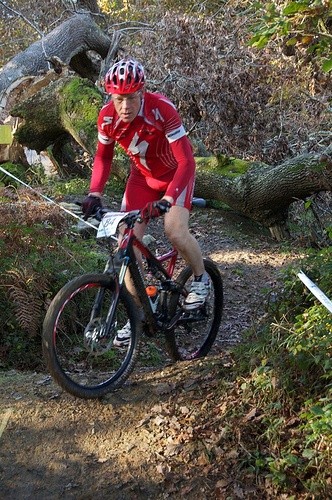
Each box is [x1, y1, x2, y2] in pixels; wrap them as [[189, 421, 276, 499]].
[[146, 285, 159, 302]]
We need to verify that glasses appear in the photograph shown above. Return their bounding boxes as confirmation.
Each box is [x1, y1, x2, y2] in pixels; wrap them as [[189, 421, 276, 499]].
[[113, 92, 141, 103]]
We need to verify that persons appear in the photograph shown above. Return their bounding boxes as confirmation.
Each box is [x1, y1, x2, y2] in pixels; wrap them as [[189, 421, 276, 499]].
[[82, 60, 213, 347]]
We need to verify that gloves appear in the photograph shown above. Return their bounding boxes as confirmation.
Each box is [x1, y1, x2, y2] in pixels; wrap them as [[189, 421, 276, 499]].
[[82, 196, 100, 218], [142, 199, 172, 224]]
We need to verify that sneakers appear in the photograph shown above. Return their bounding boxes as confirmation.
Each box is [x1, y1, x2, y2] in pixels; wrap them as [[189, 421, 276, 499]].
[[184, 282, 211, 310], [113, 319, 131, 347]]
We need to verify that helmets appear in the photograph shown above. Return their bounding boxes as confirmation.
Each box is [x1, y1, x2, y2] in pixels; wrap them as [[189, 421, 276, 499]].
[[104, 58, 146, 94]]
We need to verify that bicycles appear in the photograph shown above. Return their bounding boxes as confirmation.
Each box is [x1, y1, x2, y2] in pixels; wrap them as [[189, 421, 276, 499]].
[[41, 201, 224, 400]]
